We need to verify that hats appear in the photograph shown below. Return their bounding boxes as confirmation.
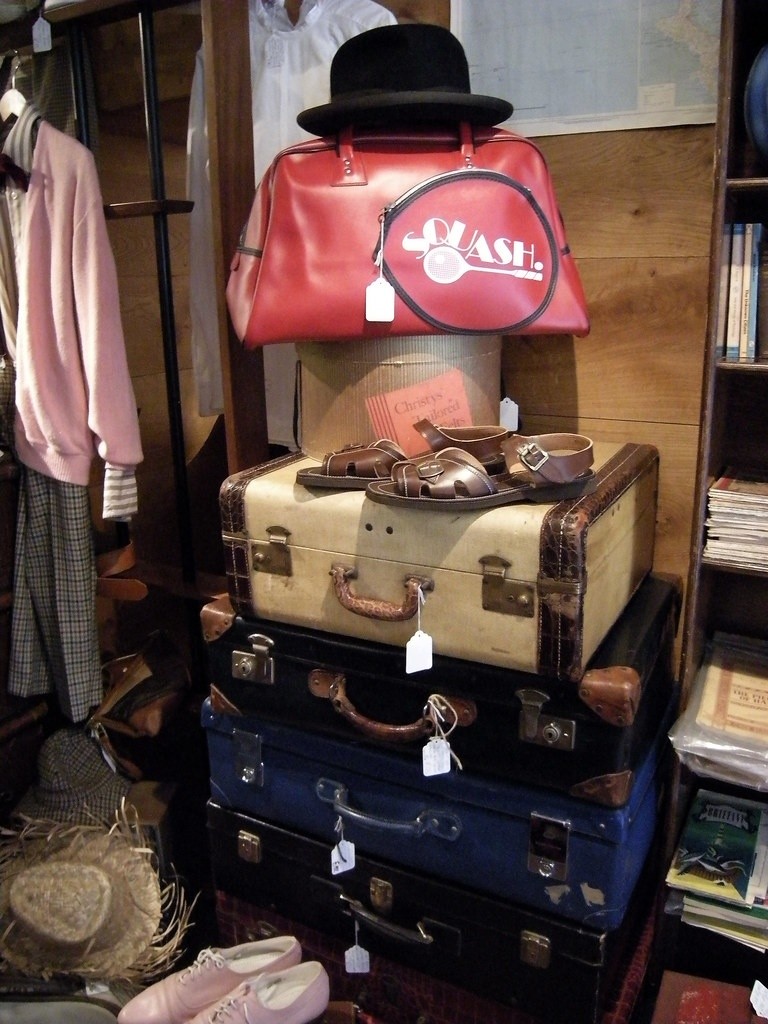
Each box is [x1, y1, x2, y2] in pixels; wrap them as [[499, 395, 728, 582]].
[[0, 796, 198, 986], [6, 727, 131, 835], [296, 23, 514, 137]]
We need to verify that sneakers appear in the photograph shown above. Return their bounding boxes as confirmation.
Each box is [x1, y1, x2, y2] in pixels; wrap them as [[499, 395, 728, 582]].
[[183, 961, 330, 1024], [115, 933, 302, 1024]]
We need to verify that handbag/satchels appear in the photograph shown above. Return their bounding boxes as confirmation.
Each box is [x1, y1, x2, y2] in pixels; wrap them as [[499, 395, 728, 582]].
[[225, 121, 587, 349], [81, 627, 197, 781]]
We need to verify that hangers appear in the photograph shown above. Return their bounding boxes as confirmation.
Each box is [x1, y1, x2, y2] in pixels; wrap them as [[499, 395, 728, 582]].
[[0, 47, 28, 121]]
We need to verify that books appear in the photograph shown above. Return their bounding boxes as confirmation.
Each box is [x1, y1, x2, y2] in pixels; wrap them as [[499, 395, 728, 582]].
[[702, 466, 768, 572], [665, 789, 768, 953], [669, 629, 768, 793], [716, 224, 768, 363]]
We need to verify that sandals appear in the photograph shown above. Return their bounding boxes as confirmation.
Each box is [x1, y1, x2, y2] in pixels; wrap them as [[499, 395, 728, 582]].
[[366, 434, 597, 512], [294, 417, 510, 489]]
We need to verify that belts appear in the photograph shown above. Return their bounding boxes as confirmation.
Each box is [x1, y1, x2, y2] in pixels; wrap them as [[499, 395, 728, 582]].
[[96, 533, 148, 602]]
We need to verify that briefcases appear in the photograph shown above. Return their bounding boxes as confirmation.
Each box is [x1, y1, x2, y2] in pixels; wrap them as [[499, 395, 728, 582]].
[[198, 571, 683, 808], [200, 797, 666, 1024], [199, 680, 681, 934], [218, 439, 662, 680]]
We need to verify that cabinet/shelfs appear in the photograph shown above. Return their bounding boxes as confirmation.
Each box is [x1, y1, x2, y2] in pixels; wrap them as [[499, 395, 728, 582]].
[[650, 1, 767, 999]]
